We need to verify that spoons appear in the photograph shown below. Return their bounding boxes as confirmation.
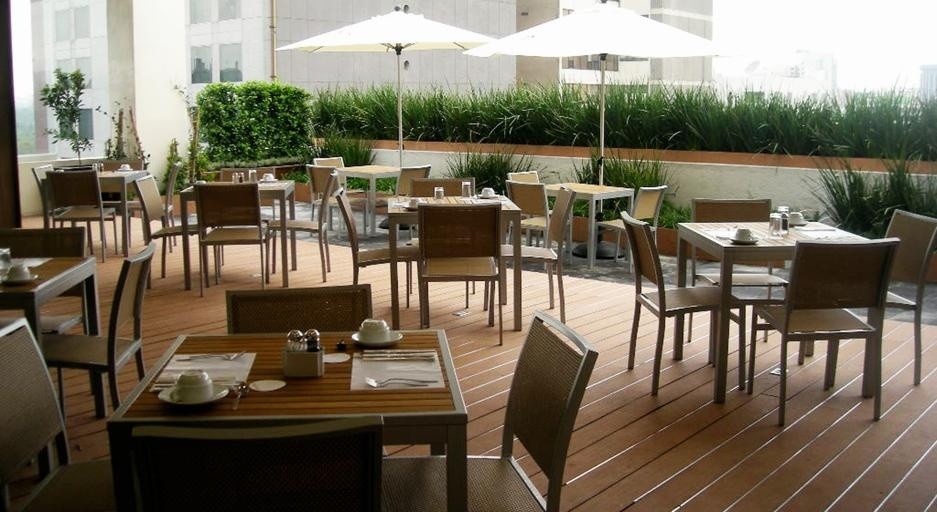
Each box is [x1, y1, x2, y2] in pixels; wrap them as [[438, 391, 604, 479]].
[[231, 381, 249, 413], [175, 350, 247, 361], [365, 380, 430, 389]]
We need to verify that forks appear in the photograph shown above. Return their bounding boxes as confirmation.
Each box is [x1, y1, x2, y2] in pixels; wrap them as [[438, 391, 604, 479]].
[[365, 375, 439, 384]]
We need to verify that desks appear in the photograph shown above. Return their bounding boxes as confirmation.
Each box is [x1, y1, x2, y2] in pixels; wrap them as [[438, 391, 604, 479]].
[[673, 218, 879, 403], [387, 194, 525, 332], [1, 254, 104, 424], [85, 170, 153, 257], [180, 180, 297, 290], [107, 325, 470, 510], [540, 181, 635, 271]]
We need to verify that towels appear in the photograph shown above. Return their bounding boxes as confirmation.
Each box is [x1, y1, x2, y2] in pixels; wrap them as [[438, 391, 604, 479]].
[[351, 347, 446, 392], [147, 351, 257, 389]]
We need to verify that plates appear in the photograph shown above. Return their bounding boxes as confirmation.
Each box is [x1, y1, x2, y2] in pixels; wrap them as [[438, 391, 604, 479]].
[[730, 236, 760, 244], [259, 178, 279, 182], [157, 382, 230, 406], [2, 273, 38, 283], [351, 331, 404, 345], [788, 222, 807, 227], [403, 205, 418, 210], [478, 195, 500, 198]]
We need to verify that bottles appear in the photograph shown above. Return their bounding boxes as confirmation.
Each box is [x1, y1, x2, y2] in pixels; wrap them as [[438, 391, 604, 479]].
[[305, 327, 319, 351], [782, 212, 790, 230], [232, 172, 240, 183], [91, 163, 98, 171], [98, 163, 104, 174], [287, 328, 304, 353], [239, 172, 245, 183]]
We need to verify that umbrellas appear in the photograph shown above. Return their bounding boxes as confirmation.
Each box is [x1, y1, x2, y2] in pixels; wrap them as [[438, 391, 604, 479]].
[[461, 2, 729, 59], [275, 8, 498, 52]]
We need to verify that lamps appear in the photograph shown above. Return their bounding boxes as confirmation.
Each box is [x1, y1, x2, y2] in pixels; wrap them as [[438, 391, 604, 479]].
[[327, 163, 402, 237]]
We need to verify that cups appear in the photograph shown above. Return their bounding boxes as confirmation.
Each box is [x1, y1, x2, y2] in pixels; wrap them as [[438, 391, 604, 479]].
[[263, 174, 274, 181], [461, 182, 472, 199], [408, 198, 423, 207], [357, 318, 391, 341], [734, 228, 753, 240], [789, 211, 804, 223], [177, 367, 213, 400], [248, 169, 258, 183], [6, 263, 31, 278], [434, 186, 444, 200], [480, 188, 495, 195]]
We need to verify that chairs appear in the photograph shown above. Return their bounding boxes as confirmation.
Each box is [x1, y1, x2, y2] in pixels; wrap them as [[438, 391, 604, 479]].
[[127, 161, 183, 253], [141, 161, 182, 253], [40, 241, 157, 420], [46, 171, 118, 263], [219, 168, 276, 219], [32, 165, 93, 255], [413, 201, 503, 345], [132, 174, 208, 287], [508, 171, 542, 218], [127, 416, 383, 510], [596, 184, 668, 276], [224, 284, 373, 334], [809, 209, 936, 386], [3, 228, 89, 396], [624, 214, 746, 395], [314, 156, 347, 230], [384, 312, 597, 511], [688, 197, 790, 346], [270, 170, 336, 282], [508, 184, 569, 272], [411, 178, 478, 295], [500, 187, 576, 325], [193, 183, 267, 297], [395, 166, 432, 241], [748, 235, 900, 428], [102, 159, 144, 247], [336, 190, 417, 309], [306, 163, 368, 239], [1, 318, 120, 511]]
